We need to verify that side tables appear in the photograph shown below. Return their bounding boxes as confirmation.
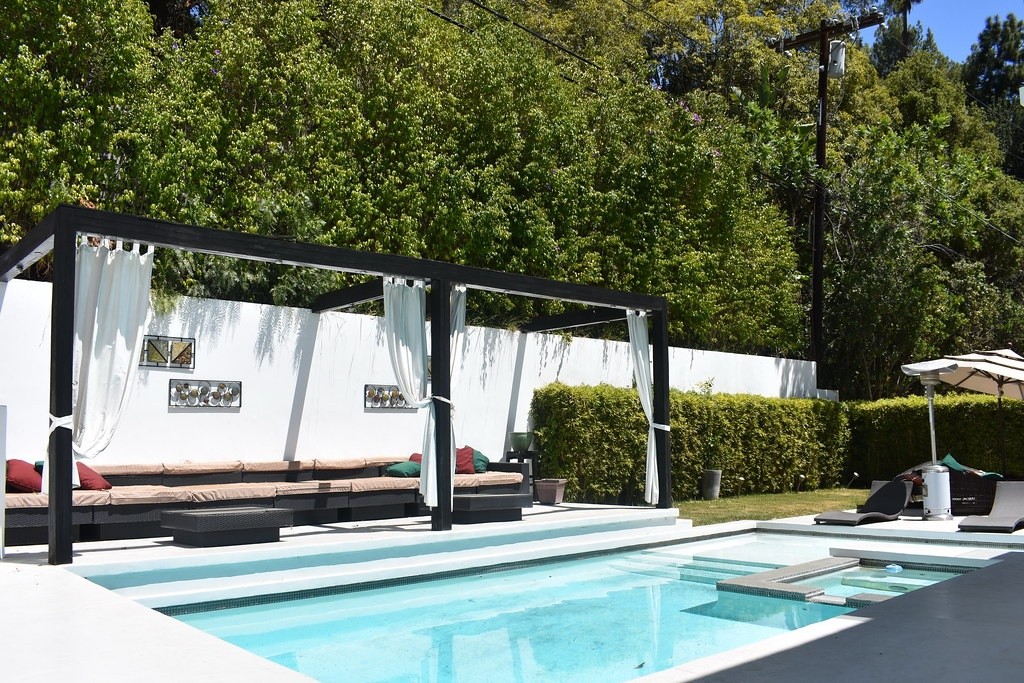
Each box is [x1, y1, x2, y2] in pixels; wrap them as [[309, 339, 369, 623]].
[[506, 451, 539, 502]]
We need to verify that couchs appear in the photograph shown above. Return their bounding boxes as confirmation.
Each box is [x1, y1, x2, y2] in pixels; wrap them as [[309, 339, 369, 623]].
[[161, 460, 276, 537], [456, 461, 530, 494], [242, 459, 352, 528], [5, 490, 111, 547], [314, 457, 420, 522], [364, 456, 479, 518], [87, 462, 193, 541]]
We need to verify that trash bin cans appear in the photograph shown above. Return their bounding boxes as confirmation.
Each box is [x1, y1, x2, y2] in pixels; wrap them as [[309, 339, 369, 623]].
[[921, 464, 953, 520]]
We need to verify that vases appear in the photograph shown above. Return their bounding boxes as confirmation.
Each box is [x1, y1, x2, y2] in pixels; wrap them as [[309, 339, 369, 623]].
[[509, 432, 533, 451], [534, 478, 568, 505], [701, 469, 722, 500]]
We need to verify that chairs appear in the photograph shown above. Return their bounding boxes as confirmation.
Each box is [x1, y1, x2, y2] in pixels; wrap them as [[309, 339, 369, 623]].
[[958, 481, 1024, 533], [813, 481, 913, 525]]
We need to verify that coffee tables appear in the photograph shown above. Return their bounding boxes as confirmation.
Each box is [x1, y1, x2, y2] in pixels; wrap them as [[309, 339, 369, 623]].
[[452, 494, 533, 524], [161, 506, 294, 548]]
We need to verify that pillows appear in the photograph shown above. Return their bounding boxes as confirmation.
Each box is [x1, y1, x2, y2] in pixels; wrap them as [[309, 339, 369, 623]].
[[455, 448, 475, 473], [465, 445, 489, 473], [410, 453, 422, 463], [35, 461, 43, 474], [77, 461, 112, 489], [386, 462, 421, 477], [7, 459, 42, 493]]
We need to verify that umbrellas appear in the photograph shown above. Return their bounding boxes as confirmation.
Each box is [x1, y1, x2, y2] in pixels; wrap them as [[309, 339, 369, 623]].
[[901, 348, 1024, 474]]
